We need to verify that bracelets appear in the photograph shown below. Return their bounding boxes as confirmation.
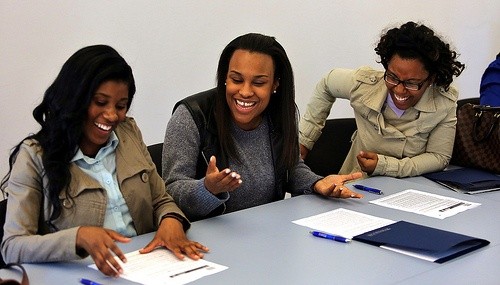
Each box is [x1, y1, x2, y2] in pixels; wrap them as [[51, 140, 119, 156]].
[[161, 215, 185, 228]]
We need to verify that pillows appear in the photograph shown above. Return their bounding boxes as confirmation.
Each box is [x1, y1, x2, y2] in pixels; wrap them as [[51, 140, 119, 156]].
[[456, 103, 500, 171]]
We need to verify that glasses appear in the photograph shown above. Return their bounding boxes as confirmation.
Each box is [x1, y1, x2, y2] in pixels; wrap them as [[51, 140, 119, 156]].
[[383, 70, 431, 91]]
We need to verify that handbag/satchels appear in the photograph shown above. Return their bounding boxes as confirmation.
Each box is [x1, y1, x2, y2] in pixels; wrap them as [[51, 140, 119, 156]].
[[450, 102, 500, 176]]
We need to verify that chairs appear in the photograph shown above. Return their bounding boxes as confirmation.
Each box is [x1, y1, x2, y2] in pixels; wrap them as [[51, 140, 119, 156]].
[[452, 97, 484, 167], [304, 118, 358, 178]]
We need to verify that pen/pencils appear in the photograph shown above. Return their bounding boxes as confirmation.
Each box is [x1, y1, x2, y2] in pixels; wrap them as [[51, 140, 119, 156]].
[[309, 231, 352, 243], [80, 278, 100, 285], [352, 184, 383, 195]]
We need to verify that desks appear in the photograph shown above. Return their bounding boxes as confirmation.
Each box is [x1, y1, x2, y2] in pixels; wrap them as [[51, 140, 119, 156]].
[[0, 175, 500, 285]]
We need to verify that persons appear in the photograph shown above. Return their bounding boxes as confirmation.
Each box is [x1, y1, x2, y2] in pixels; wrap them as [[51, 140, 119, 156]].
[[162, 33, 365, 223], [1, 44, 210, 278], [298, 20, 465, 185], [479, 52, 500, 107]]
